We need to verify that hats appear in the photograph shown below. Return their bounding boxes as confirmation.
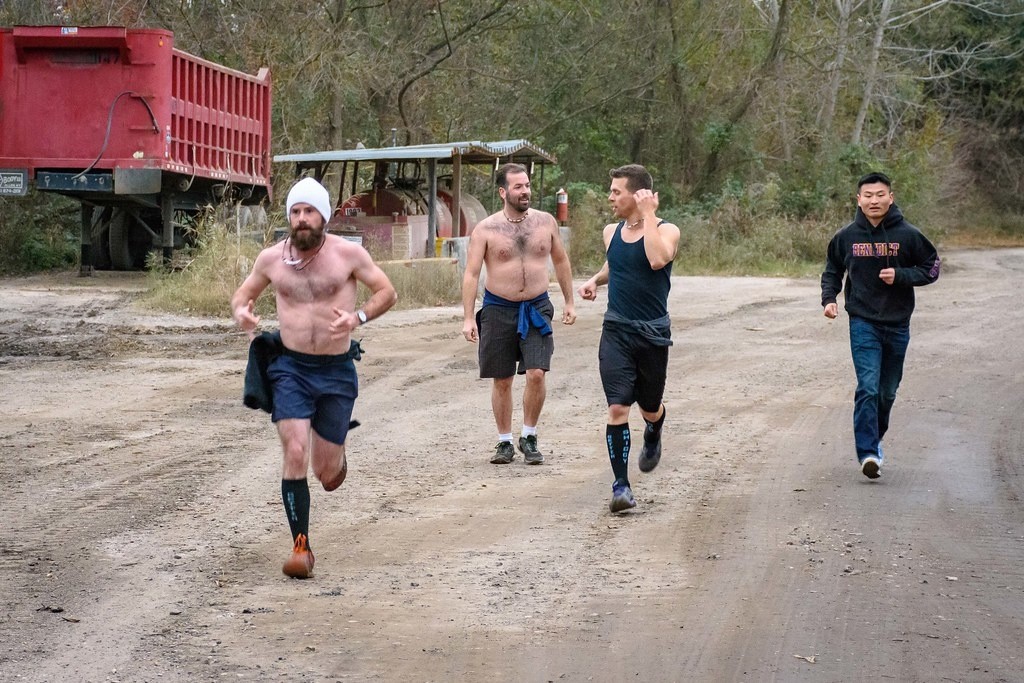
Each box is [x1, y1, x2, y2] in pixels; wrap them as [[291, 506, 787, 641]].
[[287, 177, 331, 226]]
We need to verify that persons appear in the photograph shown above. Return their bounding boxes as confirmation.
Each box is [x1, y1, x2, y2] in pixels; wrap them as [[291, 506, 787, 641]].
[[230, 176, 399, 581], [577, 164, 681, 512], [463, 164, 576, 466], [822, 171, 940, 480]]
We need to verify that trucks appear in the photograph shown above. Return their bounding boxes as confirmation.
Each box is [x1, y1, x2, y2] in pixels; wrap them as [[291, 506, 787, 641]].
[[0, 25, 275, 271]]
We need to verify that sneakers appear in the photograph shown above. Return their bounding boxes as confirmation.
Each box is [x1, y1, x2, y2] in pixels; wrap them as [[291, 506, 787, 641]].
[[517, 434, 544, 464], [639, 432, 660, 474], [861, 444, 884, 479], [491, 444, 514, 464], [282, 549, 315, 576], [610, 479, 636, 513], [321, 456, 346, 493]]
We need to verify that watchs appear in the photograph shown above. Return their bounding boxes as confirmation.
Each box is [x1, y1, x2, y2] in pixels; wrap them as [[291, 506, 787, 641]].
[[356, 309, 367, 325]]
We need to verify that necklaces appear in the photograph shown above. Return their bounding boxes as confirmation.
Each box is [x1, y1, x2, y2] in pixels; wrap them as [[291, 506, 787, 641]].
[[624, 217, 644, 229], [503, 207, 528, 223], [282, 231, 326, 271]]
